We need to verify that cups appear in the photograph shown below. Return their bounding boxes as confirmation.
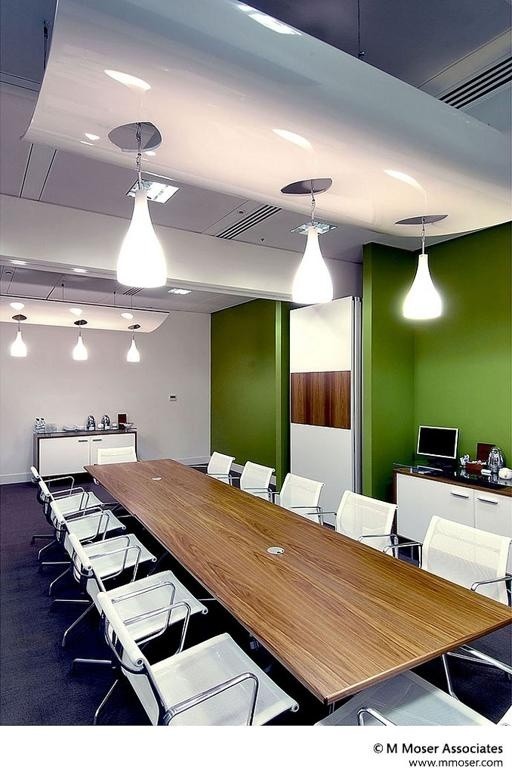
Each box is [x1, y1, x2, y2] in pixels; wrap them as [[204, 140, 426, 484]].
[[98, 424, 104, 428], [112, 423, 117, 427]]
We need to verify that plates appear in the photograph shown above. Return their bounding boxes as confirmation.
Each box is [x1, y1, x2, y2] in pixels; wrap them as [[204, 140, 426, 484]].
[[111, 428, 119, 430], [77, 428, 84, 431], [65, 428, 75, 431], [96, 428, 104, 431]]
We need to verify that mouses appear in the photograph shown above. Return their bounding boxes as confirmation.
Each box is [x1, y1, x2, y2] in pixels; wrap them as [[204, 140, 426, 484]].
[[433, 470, 442, 476]]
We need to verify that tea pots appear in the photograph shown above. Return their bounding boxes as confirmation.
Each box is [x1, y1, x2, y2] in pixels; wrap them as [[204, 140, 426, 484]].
[[101, 414, 110, 428], [87, 415, 96, 429], [488, 447, 505, 472]]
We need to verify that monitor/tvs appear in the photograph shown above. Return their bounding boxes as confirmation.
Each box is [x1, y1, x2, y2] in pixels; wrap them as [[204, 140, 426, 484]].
[[416, 426, 459, 471]]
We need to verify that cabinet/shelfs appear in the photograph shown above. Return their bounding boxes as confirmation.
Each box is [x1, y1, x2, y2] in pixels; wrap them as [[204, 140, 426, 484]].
[[34, 428, 137, 486]]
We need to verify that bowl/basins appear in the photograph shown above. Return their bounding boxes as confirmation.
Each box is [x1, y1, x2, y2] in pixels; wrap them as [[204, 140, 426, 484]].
[[124, 423, 133, 428]]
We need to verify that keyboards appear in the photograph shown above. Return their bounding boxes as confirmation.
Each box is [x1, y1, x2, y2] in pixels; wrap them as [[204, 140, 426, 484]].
[[409, 467, 434, 475]]
